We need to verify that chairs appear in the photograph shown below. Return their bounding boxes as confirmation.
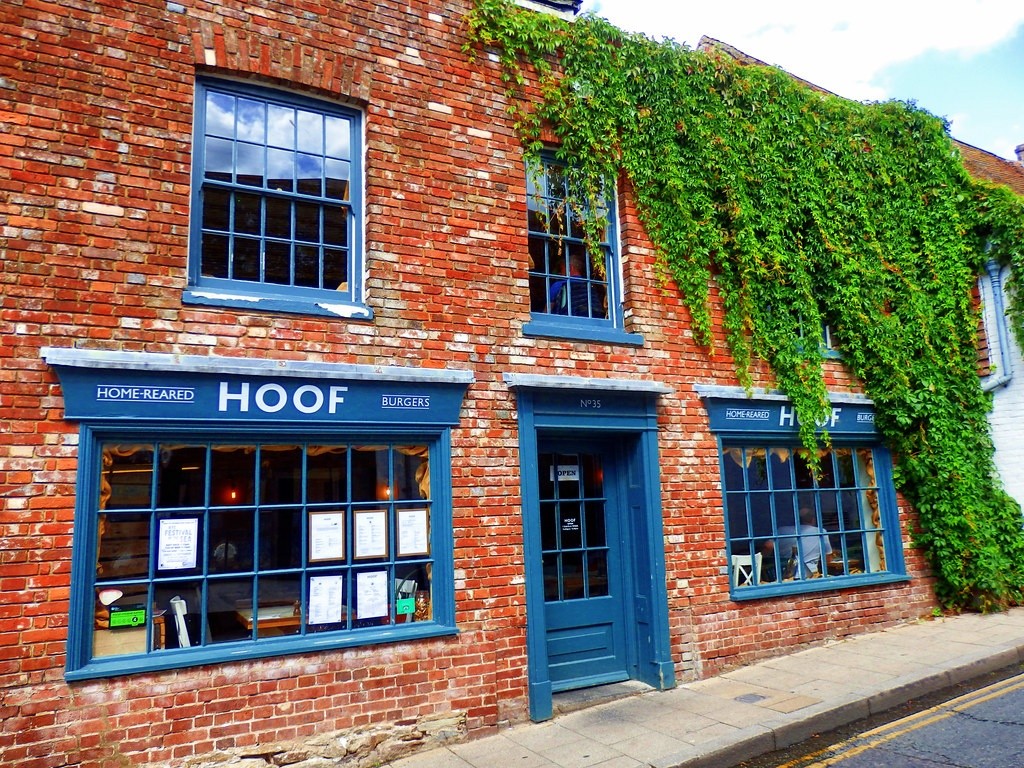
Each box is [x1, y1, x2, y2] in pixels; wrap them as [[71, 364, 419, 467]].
[[731, 546, 797, 587], [555, 282, 609, 319], [169, 595, 192, 648]]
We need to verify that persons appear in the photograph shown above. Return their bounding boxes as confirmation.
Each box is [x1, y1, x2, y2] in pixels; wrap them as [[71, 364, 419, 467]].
[[542, 253, 605, 319], [763, 507, 833, 580]]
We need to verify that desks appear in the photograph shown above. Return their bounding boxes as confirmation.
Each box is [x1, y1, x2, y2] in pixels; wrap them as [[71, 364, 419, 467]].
[[210, 585, 356, 638], [828, 556, 863, 570]]
